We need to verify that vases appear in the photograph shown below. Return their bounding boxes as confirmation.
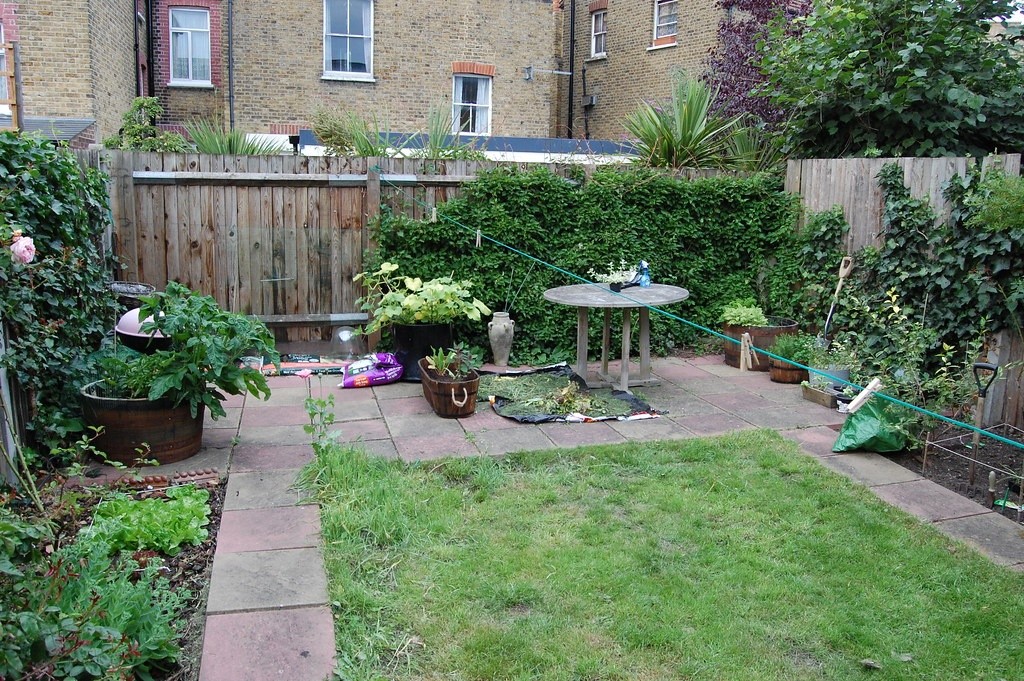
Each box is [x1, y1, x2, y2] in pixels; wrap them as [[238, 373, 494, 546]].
[[487, 311, 515, 366]]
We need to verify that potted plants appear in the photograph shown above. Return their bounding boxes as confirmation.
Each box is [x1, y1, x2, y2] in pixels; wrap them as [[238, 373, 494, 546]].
[[800, 375, 841, 408], [586, 257, 638, 293], [836, 380, 854, 410], [419, 342, 485, 419], [716, 303, 799, 371], [80, 280, 282, 468], [995, 463, 1021, 494], [808, 347, 855, 389], [348, 262, 493, 383], [766, 329, 818, 384]]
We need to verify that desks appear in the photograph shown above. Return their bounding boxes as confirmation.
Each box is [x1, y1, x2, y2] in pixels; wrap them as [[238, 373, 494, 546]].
[[544, 283, 689, 396]]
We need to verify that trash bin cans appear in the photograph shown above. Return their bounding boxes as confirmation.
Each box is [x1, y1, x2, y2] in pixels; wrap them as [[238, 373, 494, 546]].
[[103, 278, 156, 318], [113, 307, 176, 357]]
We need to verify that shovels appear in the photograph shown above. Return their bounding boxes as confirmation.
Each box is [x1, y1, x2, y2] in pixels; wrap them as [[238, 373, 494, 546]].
[[813, 256, 855, 353]]
